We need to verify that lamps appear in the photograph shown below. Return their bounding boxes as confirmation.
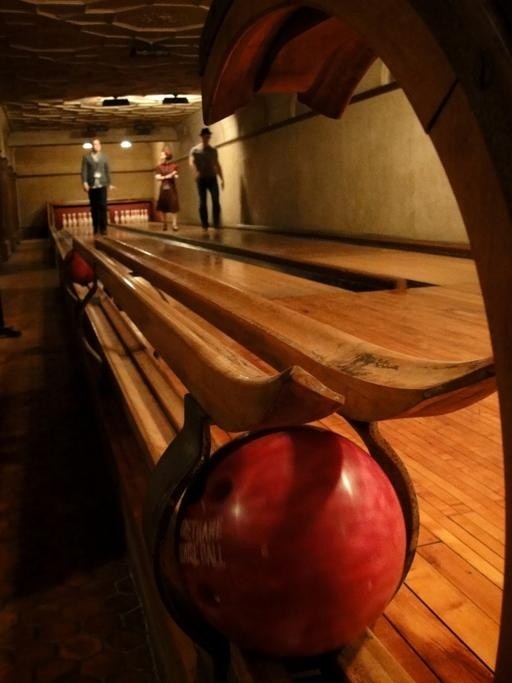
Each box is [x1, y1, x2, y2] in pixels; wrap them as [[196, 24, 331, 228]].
[[101, 94, 131, 106], [119, 125, 132, 149], [82, 126, 94, 149], [162, 91, 190, 104]]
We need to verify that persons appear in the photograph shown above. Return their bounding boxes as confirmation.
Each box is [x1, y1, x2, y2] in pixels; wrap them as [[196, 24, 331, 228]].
[[81, 138, 113, 234], [154, 149, 179, 231], [189, 129, 225, 232]]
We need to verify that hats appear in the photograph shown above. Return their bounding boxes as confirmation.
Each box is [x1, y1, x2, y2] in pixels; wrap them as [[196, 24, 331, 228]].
[[199, 126, 213, 137]]
